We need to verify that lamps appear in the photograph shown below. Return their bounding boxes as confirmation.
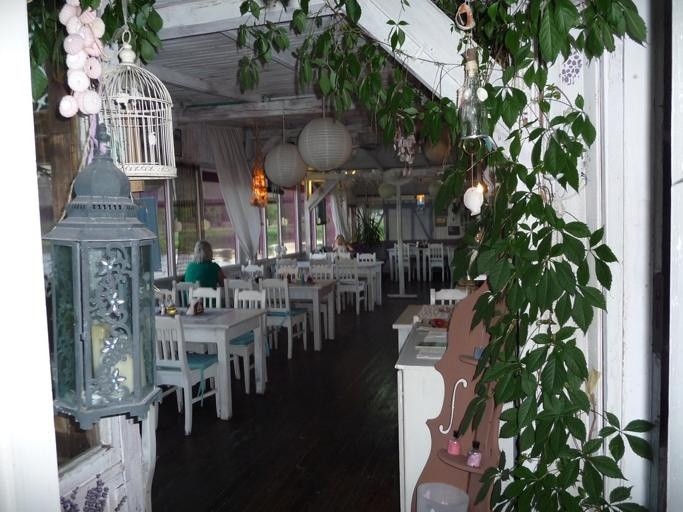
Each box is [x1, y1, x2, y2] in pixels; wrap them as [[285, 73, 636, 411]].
[[378, 182, 395, 199], [263, 112, 306, 188], [298, 95, 354, 174], [423, 134, 451, 163], [250, 124, 268, 207]]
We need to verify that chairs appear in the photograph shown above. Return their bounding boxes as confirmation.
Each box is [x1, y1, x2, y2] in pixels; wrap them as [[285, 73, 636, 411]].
[[150, 249, 385, 361], [386, 239, 456, 286], [429, 287, 469, 308], [150, 315, 221, 435], [226, 286, 270, 394]]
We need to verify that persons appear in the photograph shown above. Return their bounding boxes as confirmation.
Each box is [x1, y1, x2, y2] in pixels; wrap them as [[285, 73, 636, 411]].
[[183, 240, 224, 309], [332, 235, 354, 251]]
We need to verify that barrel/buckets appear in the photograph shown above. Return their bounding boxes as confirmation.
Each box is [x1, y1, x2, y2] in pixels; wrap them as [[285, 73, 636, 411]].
[[417, 482, 470, 512]]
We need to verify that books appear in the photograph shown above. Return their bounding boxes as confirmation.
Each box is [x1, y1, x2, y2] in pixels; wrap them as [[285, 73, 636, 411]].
[[415, 319, 450, 359]]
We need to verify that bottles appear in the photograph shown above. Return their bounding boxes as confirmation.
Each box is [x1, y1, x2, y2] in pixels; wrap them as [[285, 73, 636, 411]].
[[458, 60, 489, 141], [160, 304, 166, 316]]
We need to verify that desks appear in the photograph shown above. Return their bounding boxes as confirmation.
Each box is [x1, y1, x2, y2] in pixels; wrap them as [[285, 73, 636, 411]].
[[390, 303, 450, 512]]
